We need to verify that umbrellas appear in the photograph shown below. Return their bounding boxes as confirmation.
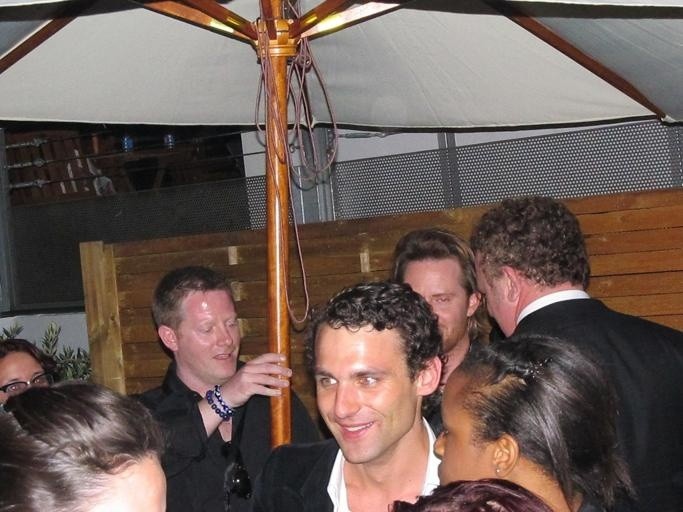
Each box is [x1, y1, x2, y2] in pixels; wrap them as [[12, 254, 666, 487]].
[[0, 0, 682, 451]]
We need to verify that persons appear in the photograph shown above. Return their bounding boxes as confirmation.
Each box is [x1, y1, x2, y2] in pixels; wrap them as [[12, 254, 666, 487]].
[[246, 281, 445, 512], [390, 225, 506, 438], [388, 475, 553, 512], [126, 264, 322, 511], [1, 339, 55, 408], [1, 381, 170, 512], [432, 333, 642, 511], [469, 194, 683, 512]]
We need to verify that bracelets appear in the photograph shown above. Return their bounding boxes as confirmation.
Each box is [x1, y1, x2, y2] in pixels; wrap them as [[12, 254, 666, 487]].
[[205, 383, 238, 422]]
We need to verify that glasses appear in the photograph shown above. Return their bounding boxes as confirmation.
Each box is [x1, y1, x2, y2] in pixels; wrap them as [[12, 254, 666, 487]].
[[0, 369, 54, 396], [222, 440, 252, 500]]
